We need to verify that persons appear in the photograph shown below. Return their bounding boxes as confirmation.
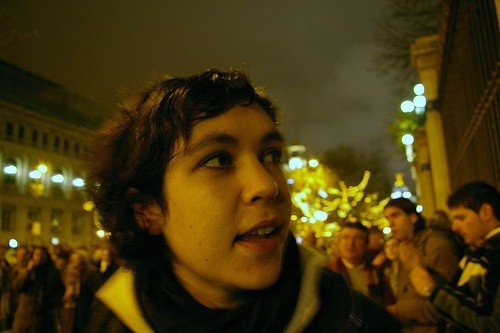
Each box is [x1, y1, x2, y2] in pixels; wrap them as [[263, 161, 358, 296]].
[[428, 207, 451, 227], [384, 197, 456, 332], [301, 229, 326, 251], [325, 222, 396, 308], [362, 225, 386, 266], [79, 68, 403, 333], [0, 241, 119, 332], [400, 180, 500, 332]]
